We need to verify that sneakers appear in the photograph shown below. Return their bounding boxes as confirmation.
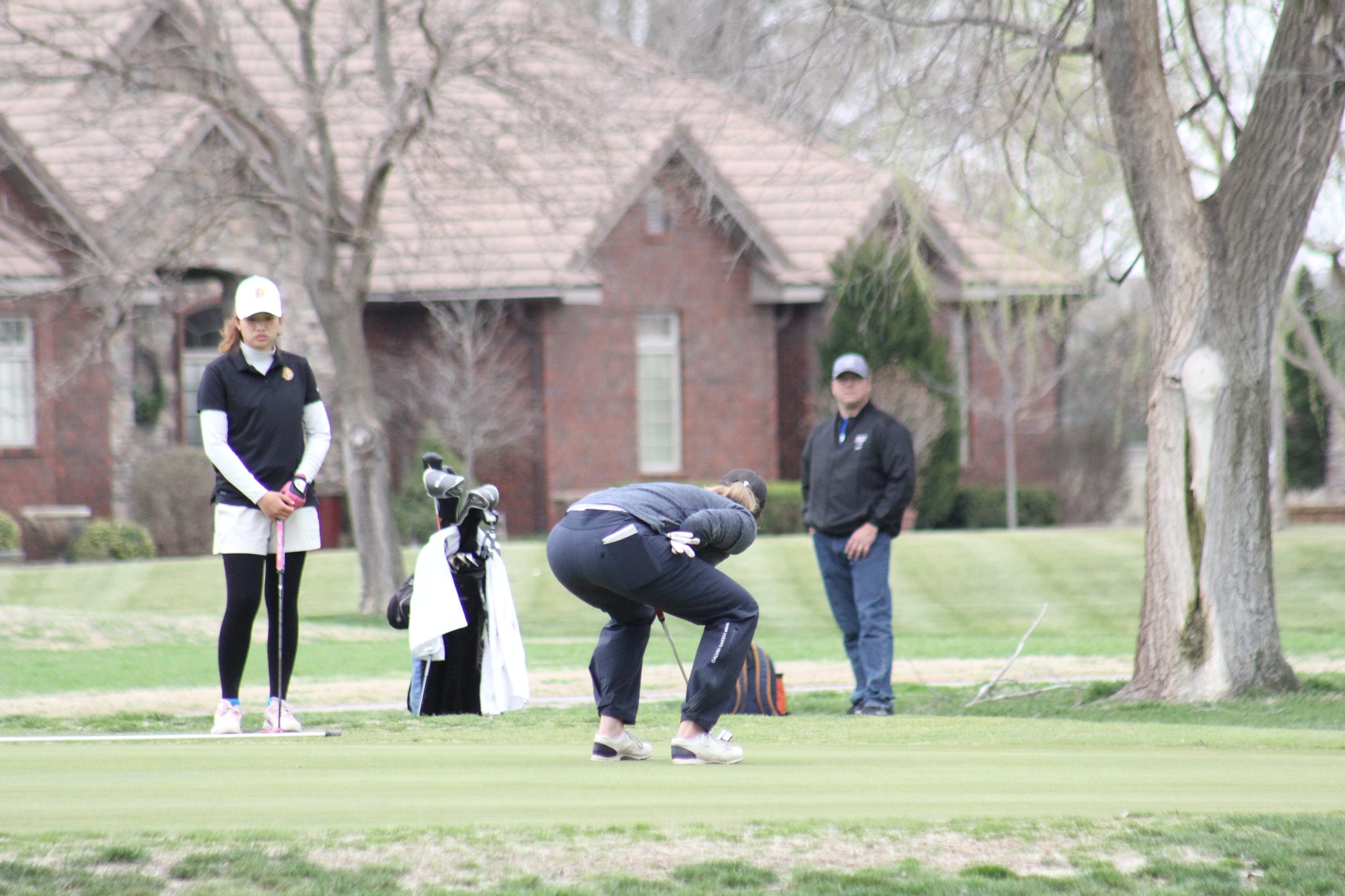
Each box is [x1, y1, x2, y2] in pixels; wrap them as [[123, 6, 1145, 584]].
[[213, 699, 242, 734], [263, 696, 301, 731], [591, 730, 652, 761], [671, 732, 745, 764]]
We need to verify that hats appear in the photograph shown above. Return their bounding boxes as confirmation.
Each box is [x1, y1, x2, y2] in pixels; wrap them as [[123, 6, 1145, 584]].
[[833, 353, 869, 378], [719, 469, 768, 520], [234, 276, 282, 319]]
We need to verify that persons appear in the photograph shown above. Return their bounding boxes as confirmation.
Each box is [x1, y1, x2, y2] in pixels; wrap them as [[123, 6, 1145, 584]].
[[546, 470, 765, 765], [801, 352, 917, 716], [196, 275, 331, 733]]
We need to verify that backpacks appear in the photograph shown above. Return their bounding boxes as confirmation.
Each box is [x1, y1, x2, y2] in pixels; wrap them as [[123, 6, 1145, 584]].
[[722, 642, 788, 716]]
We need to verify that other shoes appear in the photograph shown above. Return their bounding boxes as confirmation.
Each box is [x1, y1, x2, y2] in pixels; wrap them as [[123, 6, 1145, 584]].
[[855, 706, 892, 716], [846, 703, 862, 714]]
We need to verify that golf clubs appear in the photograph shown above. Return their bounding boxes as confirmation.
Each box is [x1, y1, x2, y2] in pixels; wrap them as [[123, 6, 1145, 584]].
[[654, 608, 733, 742], [260, 519, 285, 733], [448, 533, 501, 574]]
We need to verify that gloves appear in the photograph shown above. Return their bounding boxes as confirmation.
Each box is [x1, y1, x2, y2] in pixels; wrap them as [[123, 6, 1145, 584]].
[[666, 531, 700, 557], [280, 474, 310, 509]]
